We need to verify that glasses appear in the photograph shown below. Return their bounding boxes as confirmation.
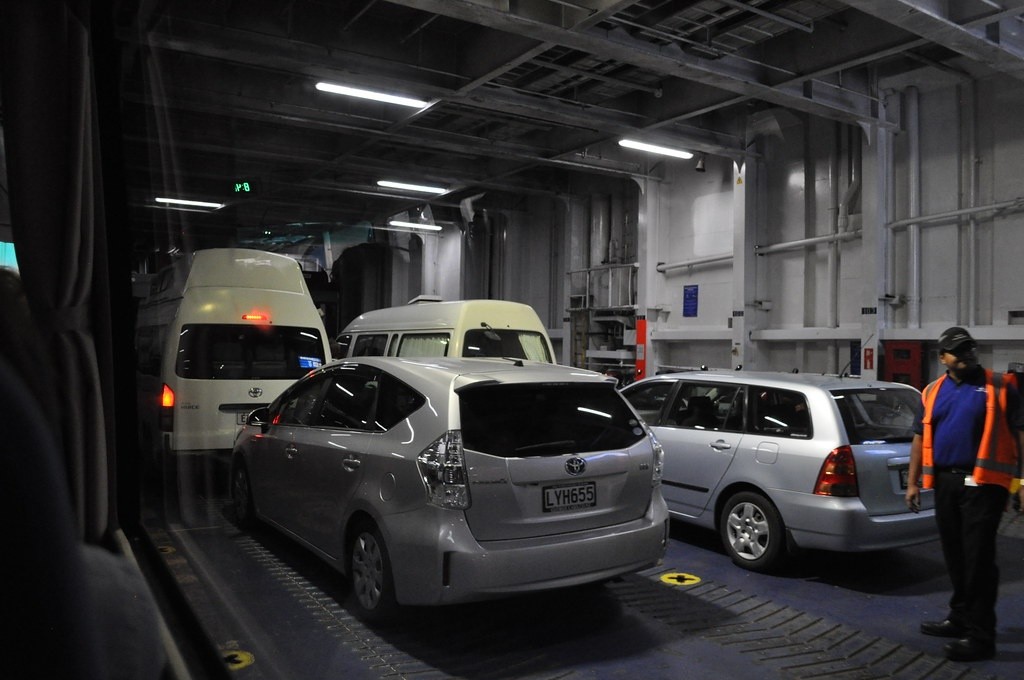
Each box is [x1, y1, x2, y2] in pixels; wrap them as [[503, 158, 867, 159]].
[[949, 342, 977, 355]]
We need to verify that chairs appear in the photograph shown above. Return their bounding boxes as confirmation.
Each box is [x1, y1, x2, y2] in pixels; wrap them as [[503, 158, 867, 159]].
[[681, 396, 719, 428]]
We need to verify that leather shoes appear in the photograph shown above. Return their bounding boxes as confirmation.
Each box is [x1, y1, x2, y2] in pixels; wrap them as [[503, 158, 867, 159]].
[[920, 618, 965, 638], [944, 636, 996, 661]]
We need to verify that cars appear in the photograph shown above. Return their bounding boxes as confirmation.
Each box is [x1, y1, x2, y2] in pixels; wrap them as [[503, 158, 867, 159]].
[[229, 356, 670, 626], [620, 373, 941, 572]]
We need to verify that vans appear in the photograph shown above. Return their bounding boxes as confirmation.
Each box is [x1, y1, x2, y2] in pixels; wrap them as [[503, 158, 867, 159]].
[[333, 294, 562, 365], [128, 248, 334, 455]]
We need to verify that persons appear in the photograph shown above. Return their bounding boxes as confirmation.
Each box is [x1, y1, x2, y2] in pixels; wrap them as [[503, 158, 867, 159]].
[[906, 327, 1024, 662], [0, 357, 167, 680]]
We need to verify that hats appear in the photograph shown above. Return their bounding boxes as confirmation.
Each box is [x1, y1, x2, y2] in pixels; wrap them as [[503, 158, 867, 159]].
[[938, 326, 976, 353]]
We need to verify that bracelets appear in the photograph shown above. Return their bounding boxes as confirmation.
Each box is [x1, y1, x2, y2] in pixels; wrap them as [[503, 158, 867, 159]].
[[1019, 479, 1024, 485]]
